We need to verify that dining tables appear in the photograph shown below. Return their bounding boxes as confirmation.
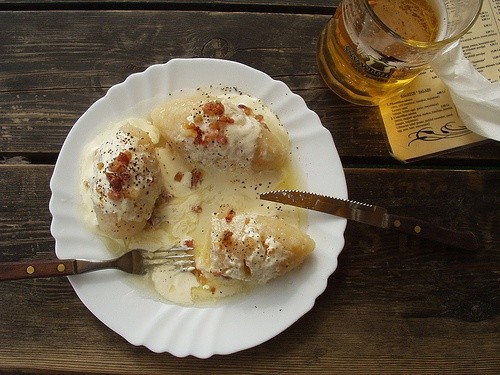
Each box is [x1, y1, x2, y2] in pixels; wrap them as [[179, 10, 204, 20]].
[[0, 0, 500, 375]]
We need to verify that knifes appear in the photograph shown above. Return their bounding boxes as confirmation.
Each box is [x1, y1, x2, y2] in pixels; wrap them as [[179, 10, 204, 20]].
[[260, 190, 477, 251]]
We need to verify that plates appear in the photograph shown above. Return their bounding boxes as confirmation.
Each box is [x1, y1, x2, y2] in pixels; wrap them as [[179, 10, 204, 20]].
[[50, 58, 348, 358]]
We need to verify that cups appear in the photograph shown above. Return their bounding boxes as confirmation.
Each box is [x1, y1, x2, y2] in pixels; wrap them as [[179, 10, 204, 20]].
[[316, 0, 483, 106]]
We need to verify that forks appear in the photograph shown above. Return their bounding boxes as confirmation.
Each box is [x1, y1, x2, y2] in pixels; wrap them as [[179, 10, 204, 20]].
[[0, 246, 195, 281]]
